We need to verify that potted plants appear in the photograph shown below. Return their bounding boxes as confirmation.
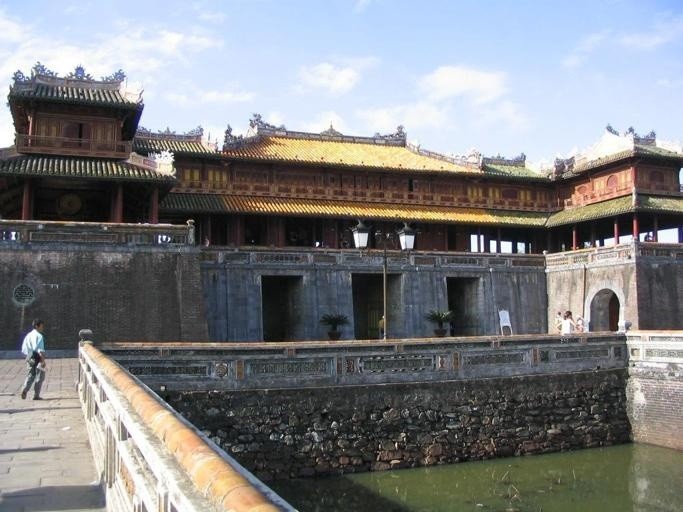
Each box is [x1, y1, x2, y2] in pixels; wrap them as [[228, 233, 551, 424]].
[[423, 307, 455, 336], [321, 312, 348, 339]]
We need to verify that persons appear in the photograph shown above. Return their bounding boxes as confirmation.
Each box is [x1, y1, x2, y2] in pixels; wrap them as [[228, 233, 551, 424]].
[[377, 314, 384, 336], [555, 310, 576, 335], [19, 316, 49, 401], [553, 312, 562, 332]]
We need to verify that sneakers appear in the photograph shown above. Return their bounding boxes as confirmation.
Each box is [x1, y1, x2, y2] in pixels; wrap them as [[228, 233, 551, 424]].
[[33, 396, 42, 399], [21, 391, 26, 398]]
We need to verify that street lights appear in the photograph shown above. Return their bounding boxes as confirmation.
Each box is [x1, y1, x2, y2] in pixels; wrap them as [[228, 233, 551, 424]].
[[350, 217, 417, 344]]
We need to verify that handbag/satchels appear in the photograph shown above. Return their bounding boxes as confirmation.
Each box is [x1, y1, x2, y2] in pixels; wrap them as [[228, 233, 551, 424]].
[[26, 350, 41, 368]]
